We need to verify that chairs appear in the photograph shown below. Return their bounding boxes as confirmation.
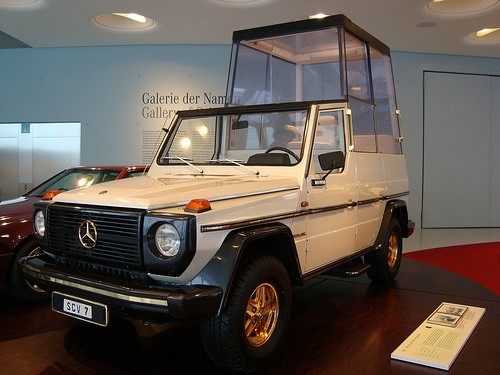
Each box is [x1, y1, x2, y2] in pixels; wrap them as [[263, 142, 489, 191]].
[[102, 175, 116, 183], [287, 116, 339, 149]]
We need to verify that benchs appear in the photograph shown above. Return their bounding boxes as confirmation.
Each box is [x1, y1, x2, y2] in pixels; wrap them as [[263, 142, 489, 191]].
[[353, 134, 394, 154]]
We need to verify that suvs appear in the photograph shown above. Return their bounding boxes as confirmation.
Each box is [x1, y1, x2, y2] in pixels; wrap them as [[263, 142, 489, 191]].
[[20, 13, 418, 375]]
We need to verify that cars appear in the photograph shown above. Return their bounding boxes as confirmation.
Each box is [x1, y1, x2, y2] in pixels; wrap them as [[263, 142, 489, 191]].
[[0, 164, 150, 304]]
